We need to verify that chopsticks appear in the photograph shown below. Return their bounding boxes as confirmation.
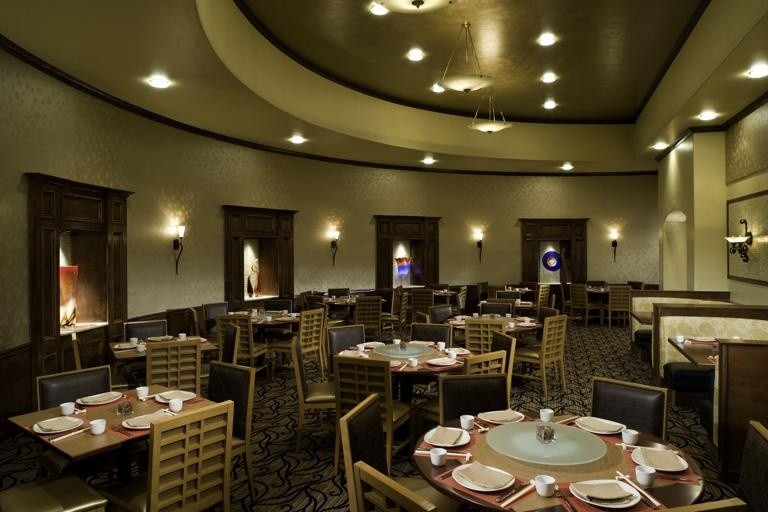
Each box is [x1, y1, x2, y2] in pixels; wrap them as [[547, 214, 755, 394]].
[[413, 450, 472, 458], [616, 470, 660, 507], [48, 426, 91, 443], [473, 421, 484, 431], [500, 484, 535, 508], [554, 415, 578, 424]]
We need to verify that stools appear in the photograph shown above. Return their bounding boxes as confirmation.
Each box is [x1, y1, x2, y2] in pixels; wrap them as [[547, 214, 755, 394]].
[[1, 475, 109, 512]]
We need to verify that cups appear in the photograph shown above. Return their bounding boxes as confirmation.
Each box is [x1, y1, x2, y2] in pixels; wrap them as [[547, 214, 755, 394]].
[[114, 332, 206, 353], [326, 294, 359, 304], [439, 288, 447, 295], [587, 285, 604, 293], [59, 402, 75, 415], [620, 428, 641, 446], [458, 414, 475, 430], [429, 446, 448, 466], [227, 308, 300, 323], [169, 398, 184, 412], [135, 386, 148, 399], [534, 474, 557, 498], [539, 409, 554, 423], [507, 285, 528, 305], [633, 465, 658, 487], [87, 419, 106, 436], [675, 335, 684, 343]]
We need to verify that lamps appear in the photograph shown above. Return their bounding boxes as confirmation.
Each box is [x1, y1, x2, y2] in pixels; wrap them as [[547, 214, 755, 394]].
[[172, 224, 184, 274], [468, 88, 512, 134], [723, 218, 752, 263], [475, 227, 484, 263], [610, 227, 617, 262], [377, 0, 451, 14], [436, 20, 493, 91], [330, 230, 342, 266]]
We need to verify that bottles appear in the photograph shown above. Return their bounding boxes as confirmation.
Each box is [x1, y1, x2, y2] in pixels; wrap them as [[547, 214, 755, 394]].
[[117, 394, 133, 417]]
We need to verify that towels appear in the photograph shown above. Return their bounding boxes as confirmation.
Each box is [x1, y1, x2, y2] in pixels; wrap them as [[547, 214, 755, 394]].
[[123, 413, 157, 428], [427, 424, 463, 445], [639, 446, 686, 472], [35, 416, 82, 432], [457, 459, 516, 491], [568, 480, 634, 505], [477, 408, 522, 423]]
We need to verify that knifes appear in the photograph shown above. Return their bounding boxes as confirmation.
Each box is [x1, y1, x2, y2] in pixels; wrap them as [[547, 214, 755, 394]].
[[493, 482, 529, 501], [552, 481, 577, 511]]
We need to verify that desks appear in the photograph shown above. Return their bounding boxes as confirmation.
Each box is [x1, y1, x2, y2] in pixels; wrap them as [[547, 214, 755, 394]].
[[223, 309, 301, 368], [113, 336, 215, 384], [336, 341, 472, 402]]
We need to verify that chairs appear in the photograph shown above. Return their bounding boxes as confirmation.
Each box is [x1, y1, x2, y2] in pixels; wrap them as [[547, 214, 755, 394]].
[[96, 400, 234, 510], [491, 330, 517, 380], [436, 372, 510, 425], [270, 308, 325, 383], [413, 352, 505, 426], [589, 373, 669, 443], [123, 320, 168, 341], [339, 392, 465, 512], [146, 338, 202, 396], [38, 365, 112, 411], [166, 308, 218, 355], [330, 353, 413, 477], [263, 279, 661, 336], [288, 335, 336, 454], [515, 316, 569, 394], [326, 323, 367, 354], [409, 322, 452, 348], [464, 319, 507, 353], [354, 460, 438, 512], [207, 360, 257, 509], [214, 313, 271, 380]]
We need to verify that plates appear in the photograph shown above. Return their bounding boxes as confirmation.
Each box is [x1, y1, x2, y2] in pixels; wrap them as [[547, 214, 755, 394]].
[[75, 391, 123, 406], [30, 416, 84, 436], [154, 388, 196, 404], [567, 478, 640, 510], [423, 426, 470, 447], [337, 338, 470, 365], [627, 448, 689, 473], [450, 464, 516, 490], [477, 410, 525, 425], [450, 313, 535, 328], [688, 337, 714, 342], [121, 413, 172, 430]]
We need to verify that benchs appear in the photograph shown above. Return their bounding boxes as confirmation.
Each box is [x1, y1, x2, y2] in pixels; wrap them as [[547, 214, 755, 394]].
[[628, 286, 766, 512]]
